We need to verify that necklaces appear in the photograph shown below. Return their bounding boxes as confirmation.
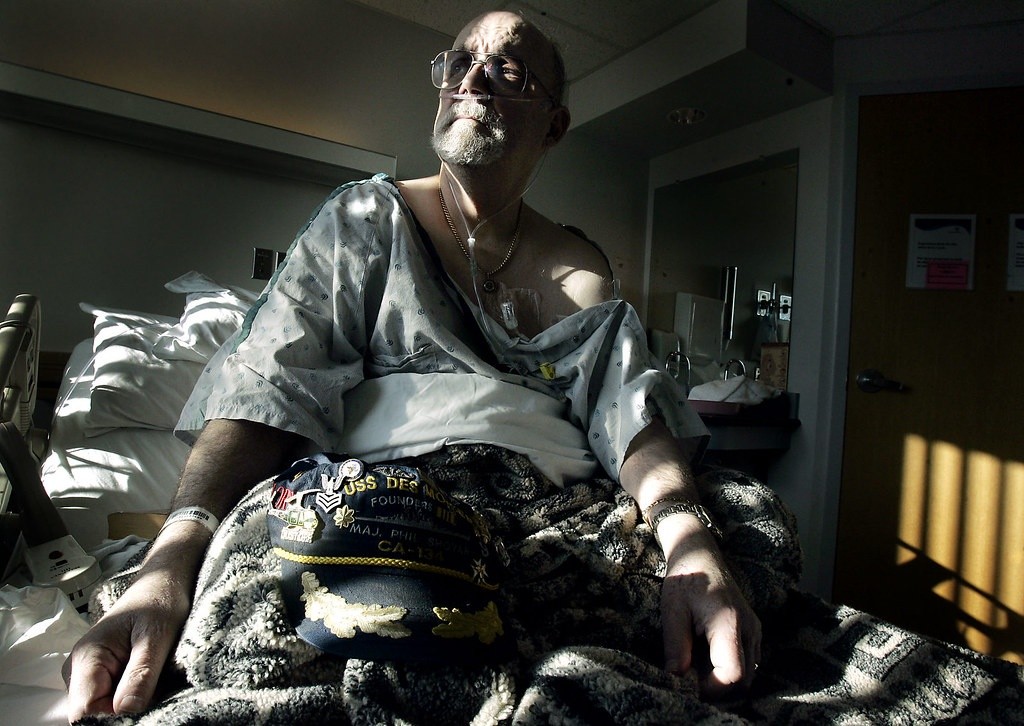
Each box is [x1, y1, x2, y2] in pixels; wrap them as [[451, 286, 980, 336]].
[[438, 188, 524, 294]]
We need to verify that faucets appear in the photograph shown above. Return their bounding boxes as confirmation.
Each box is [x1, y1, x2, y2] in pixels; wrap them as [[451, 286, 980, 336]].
[[724, 358, 747, 380], [665, 351, 691, 393]]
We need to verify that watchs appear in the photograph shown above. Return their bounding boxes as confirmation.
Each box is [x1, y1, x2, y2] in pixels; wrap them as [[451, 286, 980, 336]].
[[652, 505, 719, 548]]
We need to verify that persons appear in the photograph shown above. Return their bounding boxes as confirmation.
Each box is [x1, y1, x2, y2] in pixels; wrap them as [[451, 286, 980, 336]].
[[63, 12, 763, 726]]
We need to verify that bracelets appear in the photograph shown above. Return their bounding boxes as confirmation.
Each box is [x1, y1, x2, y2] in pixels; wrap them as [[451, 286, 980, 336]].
[[157, 506, 219, 535], [643, 497, 700, 523]]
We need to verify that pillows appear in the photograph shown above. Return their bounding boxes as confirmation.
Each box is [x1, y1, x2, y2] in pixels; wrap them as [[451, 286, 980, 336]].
[[80, 301, 203, 438], [150, 270, 261, 366]]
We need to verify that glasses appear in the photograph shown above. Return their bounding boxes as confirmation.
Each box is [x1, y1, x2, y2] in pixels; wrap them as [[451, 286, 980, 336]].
[[430, 49, 559, 104]]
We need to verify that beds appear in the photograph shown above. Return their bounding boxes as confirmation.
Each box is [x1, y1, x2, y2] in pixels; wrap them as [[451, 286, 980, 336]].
[[0, 273, 257, 726]]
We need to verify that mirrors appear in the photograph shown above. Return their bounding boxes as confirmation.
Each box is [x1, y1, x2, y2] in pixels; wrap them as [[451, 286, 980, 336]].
[[642, 147, 800, 389]]
